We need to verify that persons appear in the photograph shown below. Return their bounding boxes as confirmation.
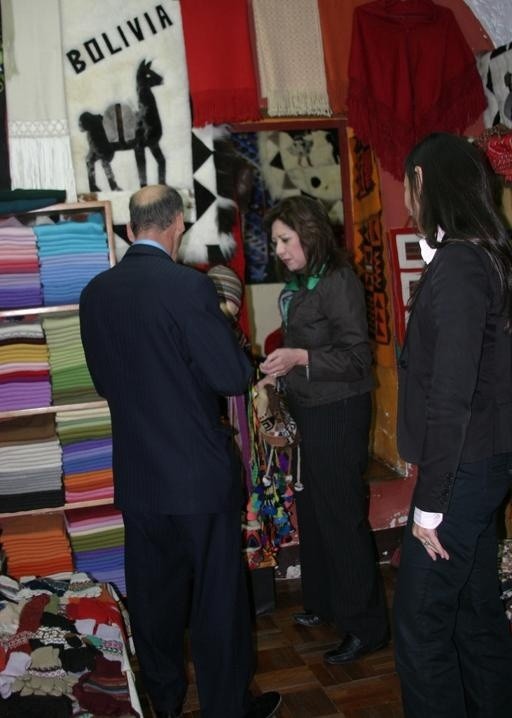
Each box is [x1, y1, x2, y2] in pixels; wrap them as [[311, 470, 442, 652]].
[[78, 183, 284, 718], [257, 192, 392, 664], [391, 129, 512, 718]]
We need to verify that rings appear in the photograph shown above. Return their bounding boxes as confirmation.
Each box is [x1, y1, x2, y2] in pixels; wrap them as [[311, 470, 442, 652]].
[[423, 541, 430, 547], [273, 373, 277, 378]]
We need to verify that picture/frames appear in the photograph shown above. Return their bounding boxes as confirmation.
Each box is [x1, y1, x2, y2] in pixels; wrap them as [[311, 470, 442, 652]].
[[389, 227, 428, 349]]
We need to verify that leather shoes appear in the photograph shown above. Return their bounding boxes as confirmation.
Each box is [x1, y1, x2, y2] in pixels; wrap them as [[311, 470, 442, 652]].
[[292, 611, 323, 626], [227, 692, 282, 718], [322, 633, 377, 665]]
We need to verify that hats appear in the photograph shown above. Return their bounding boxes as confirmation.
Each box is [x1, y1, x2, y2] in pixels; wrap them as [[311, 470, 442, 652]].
[[205, 264, 244, 307], [256, 384, 301, 447]]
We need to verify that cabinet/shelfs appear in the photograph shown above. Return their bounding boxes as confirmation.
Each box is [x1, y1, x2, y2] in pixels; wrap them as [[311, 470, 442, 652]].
[[0, 201, 117, 518]]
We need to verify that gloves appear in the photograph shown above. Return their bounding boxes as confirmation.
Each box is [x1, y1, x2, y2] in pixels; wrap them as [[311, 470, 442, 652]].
[[0, 570, 140, 718]]
